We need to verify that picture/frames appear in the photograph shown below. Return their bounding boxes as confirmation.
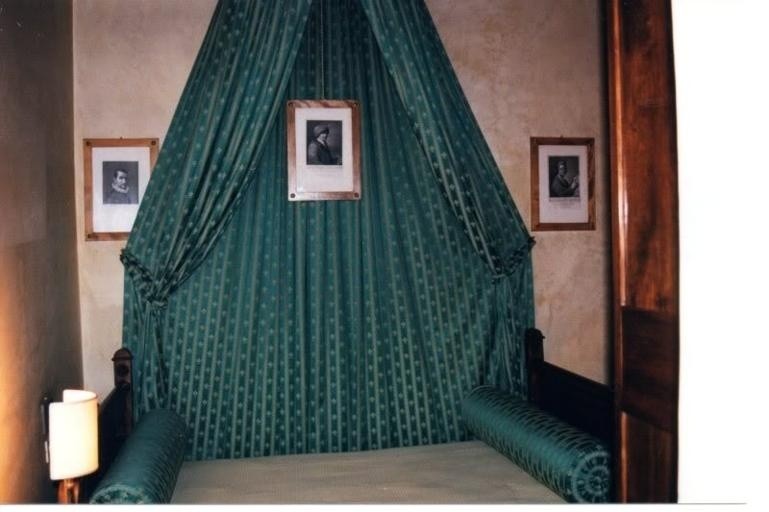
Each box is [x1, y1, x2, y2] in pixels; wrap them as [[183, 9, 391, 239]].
[[81, 136, 161, 243], [284, 97, 362, 202], [529, 134, 597, 234]]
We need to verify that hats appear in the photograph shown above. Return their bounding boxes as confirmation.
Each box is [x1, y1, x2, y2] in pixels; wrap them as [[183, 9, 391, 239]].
[[314, 125, 328, 137]]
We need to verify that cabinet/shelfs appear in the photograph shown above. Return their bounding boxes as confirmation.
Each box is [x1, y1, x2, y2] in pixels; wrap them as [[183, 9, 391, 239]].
[[597, 1, 679, 504]]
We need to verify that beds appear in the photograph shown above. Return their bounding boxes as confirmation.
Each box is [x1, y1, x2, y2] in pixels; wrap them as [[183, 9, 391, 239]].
[[44, 328, 619, 504]]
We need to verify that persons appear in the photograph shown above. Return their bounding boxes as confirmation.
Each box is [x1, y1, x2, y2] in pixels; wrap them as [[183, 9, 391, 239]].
[[551, 160, 579, 197], [308, 124, 338, 165], [105, 167, 131, 203]]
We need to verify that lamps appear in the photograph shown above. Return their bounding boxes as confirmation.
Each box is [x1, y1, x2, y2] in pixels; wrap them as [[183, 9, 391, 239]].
[[44, 388, 100, 504]]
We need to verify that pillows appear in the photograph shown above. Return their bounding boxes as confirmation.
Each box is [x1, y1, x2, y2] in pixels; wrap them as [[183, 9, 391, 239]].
[[459, 382, 614, 504], [91, 406, 188, 504]]
